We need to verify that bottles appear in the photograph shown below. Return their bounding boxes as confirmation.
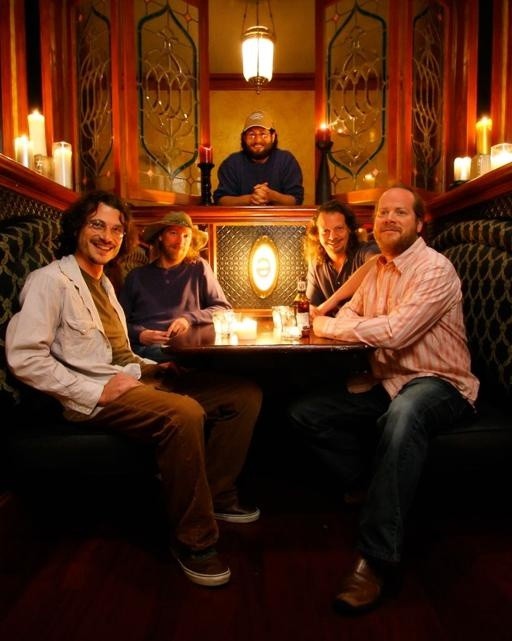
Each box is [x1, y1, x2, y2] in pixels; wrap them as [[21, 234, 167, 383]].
[[293, 281, 312, 337]]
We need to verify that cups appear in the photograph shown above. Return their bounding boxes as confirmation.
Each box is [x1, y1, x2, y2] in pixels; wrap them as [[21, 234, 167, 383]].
[[212, 310, 259, 339], [271, 305, 296, 340], [452, 144, 511, 179], [51, 141, 73, 188]]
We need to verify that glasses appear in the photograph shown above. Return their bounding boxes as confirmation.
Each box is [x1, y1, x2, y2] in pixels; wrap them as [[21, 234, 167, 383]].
[[84, 220, 125, 237]]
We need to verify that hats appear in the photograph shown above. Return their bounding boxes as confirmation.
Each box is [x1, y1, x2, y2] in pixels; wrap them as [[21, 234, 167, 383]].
[[142, 210, 208, 252], [242, 110, 276, 132]]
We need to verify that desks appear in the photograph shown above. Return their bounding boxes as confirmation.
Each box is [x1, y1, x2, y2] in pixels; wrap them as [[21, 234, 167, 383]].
[[172, 310, 378, 355]]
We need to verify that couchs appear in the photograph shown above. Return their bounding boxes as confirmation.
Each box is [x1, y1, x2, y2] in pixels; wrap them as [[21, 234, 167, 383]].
[[331, 218, 512, 597], [1, 212, 180, 559]]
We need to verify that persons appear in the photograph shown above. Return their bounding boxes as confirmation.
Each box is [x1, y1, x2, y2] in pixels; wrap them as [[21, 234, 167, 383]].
[[287, 180, 482, 613], [213, 111, 304, 206], [118, 210, 233, 364], [3, 190, 261, 587], [301, 198, 381, 317]]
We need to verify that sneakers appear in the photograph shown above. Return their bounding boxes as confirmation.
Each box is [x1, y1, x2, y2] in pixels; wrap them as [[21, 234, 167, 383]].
[[213, 502, 261, 525], [169, 538, 232, 587]]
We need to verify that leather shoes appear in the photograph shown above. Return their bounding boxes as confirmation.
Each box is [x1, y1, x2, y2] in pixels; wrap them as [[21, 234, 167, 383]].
[[332, 554, 394, 611]]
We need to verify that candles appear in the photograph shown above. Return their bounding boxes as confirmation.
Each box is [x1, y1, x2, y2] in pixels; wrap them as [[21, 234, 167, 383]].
[[453, 118, 512, 182], [197, 142, 215, 163], [315, 120, 331, 142], [14, 107, 74, 189]]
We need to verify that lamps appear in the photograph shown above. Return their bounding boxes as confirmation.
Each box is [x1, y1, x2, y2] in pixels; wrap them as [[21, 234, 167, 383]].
[[239, 1, 275, 96]]
